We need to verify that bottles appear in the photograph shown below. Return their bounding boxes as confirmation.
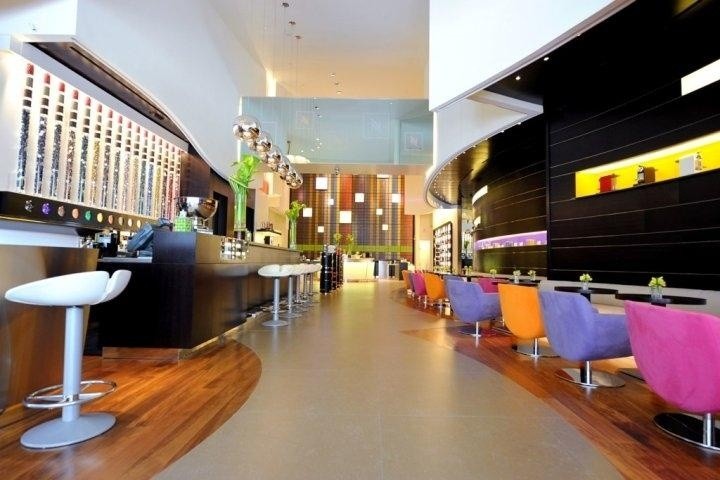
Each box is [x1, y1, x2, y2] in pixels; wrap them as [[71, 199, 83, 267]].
[[694, 151, 704, 170]]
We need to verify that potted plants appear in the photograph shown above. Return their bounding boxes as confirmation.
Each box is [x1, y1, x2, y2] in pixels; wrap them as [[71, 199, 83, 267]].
[[227, 154, 259, 232], [285, 200, 306, 249]]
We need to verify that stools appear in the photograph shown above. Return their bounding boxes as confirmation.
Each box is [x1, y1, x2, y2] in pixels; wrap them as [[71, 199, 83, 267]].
[[3, 267, 135, 451], [255, 258, 325, 328]]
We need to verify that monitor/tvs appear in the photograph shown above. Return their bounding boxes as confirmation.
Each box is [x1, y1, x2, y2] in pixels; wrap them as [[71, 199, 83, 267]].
[[126, 223, 173, 252]]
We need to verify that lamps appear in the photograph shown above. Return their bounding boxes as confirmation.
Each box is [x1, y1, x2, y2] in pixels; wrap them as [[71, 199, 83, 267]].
[[231, 0, 305, 191]]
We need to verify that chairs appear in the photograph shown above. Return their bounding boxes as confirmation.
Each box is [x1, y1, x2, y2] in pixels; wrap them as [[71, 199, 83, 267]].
[[624, 300, 720, 452], [498, 284, 632, 388], [402, 270, 512, 338]]
